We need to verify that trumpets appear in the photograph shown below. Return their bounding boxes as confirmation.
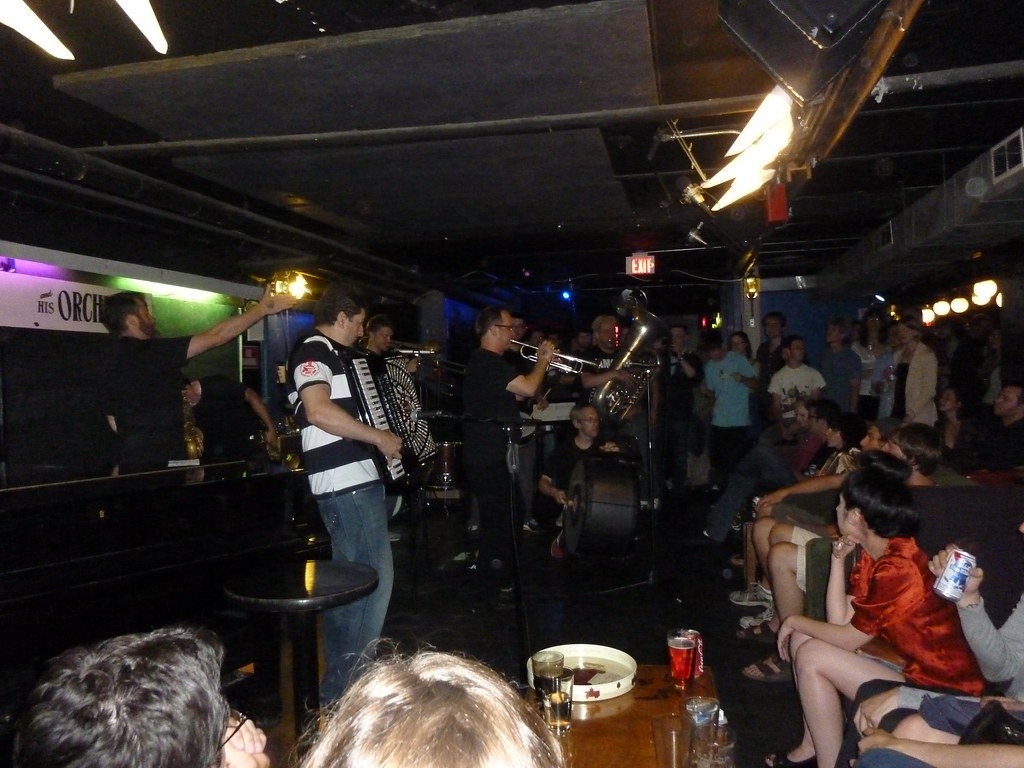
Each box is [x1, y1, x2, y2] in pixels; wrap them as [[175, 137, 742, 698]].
[[508, 337, 606, 379]]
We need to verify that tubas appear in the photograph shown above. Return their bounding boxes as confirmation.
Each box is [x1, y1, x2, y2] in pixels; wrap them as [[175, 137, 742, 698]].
[[581, 306, 675, 427]]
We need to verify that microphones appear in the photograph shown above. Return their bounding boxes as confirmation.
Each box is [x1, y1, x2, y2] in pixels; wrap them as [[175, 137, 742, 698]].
[[622, 358, 659, 367]]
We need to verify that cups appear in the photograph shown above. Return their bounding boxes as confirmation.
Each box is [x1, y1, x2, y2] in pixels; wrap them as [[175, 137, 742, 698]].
[[689, 724, 736, 768], [679, 696, 720, 766], [666, 629, 698, 689], [651, 714, 694, 768], [532, 650, 564, 713], [540, 665, 574, 734]]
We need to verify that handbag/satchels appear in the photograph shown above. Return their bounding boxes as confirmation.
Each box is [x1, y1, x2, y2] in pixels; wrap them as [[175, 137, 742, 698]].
[[956, 702, 1024, 745]]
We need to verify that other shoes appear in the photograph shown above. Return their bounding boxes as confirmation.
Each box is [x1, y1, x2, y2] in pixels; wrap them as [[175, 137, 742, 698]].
[[765, 747, 817, 768], [730, 554, 746, 566], [467, 522, 483, 540], [524, 520, 541, 532], [496, 586, 513, 610]]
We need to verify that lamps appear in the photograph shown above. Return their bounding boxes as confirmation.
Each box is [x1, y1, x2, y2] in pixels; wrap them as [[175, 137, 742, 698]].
[[743, 267, 761, 318]]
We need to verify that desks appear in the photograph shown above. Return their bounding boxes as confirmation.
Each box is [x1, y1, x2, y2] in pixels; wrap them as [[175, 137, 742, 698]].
[[220, 558, 380, 759], [525, 657, 731, 768]]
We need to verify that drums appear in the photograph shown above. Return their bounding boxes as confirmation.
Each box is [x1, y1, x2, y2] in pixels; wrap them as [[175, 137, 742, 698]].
[[427, 440, 463, 491], [563, 455, 653, 566]]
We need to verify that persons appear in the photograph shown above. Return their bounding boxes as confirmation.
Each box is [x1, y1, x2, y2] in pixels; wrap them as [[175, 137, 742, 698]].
[[853, 696, 1024, 768], [0, 629, 270, 768], [301, 652, 565, 768], [834, 523, 1024, 768], [459, 308, 1024, 679], [101, 284, 296, 625], [765, 467, 986, 768], [287, 284, 404, 711], [181, 374, 277, 481], [366, 315, 417, 372]]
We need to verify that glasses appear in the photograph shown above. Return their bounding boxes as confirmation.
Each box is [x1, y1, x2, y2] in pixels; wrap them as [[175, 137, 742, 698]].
[[493, 324, 514, 331], [731, 341, 745, 346], [216, 709, 248, 753]]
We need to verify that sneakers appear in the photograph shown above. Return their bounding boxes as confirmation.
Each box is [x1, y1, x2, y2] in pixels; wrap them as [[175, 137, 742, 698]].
[[738, 602, 777, 628], [729, 583, 776, 608]]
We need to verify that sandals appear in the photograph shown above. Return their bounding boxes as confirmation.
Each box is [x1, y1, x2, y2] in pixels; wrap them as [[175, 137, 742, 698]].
[[742, 649, 795, 684], [735, 622, 778, 644]]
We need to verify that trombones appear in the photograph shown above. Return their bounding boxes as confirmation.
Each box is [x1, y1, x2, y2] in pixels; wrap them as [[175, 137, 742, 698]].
[[355, 334, 472, 378]]
